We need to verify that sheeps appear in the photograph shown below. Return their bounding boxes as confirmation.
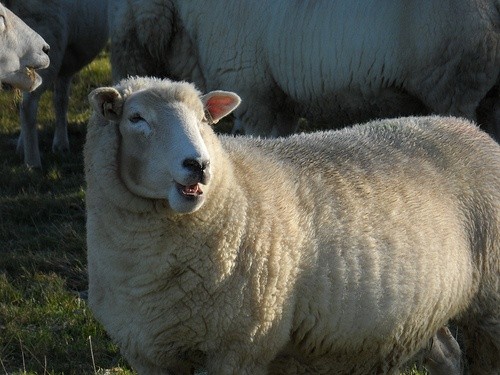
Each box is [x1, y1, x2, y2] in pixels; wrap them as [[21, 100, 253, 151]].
[[0, 0, 500, 375]]
